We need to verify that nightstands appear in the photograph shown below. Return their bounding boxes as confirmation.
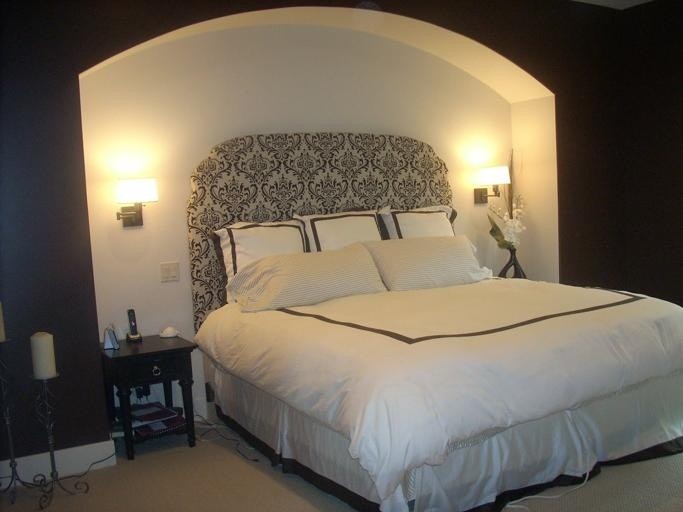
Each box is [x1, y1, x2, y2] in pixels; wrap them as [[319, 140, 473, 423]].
[[99, 333, 198, 460]]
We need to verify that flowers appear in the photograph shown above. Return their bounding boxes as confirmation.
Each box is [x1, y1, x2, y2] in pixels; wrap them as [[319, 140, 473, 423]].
[[487, 147, 526, 250]]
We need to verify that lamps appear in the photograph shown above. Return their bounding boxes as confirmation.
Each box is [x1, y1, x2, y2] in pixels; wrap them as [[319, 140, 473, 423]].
[[0, 300, 47, 506], [114, 178, 159, 227], [474, 166, 511, 204], [30, 332, 89, 509]]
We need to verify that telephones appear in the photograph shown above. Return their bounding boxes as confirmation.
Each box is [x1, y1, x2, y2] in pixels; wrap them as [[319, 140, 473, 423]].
[[127, 309, 142, 343]]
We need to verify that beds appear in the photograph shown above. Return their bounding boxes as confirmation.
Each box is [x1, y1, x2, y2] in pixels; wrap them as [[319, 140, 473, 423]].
[[185, 132, 682, 510]]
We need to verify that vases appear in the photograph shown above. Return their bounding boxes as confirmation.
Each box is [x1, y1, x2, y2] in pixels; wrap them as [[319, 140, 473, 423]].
[[498, 248, 527, 279]]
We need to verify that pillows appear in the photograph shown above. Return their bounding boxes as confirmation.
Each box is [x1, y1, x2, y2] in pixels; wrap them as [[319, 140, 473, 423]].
[[212, 218, 311, 305], [363, 234, 492, 290], [375, 204, 458, 238], [225, 240, 388, 312], [293, 208, 386, 252]]
